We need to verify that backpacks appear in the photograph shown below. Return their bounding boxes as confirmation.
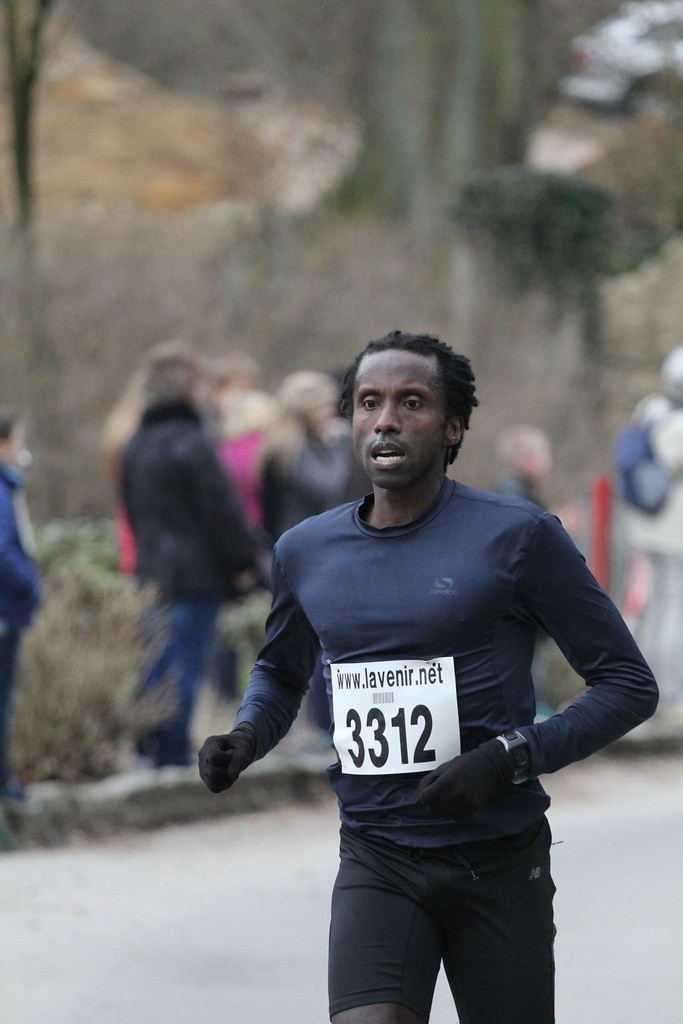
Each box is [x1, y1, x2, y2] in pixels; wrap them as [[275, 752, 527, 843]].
[[615, 403, 673, 516]]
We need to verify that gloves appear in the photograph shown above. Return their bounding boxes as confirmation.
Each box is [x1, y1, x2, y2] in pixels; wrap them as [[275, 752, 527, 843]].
[[412, 739, 515, 822], [198, 727, 257, 793]]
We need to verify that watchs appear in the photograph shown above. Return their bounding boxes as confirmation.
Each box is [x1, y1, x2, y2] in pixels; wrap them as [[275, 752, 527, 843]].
[[496, 731, 531, 784]]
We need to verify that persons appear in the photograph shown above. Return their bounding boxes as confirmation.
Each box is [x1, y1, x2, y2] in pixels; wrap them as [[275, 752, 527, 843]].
[[496, 425, 554, 509], [100, 341, 372, 770], [0, 411, 43, 804], [618, 346, 683, 702], [198, 332, 659, 1024]]
